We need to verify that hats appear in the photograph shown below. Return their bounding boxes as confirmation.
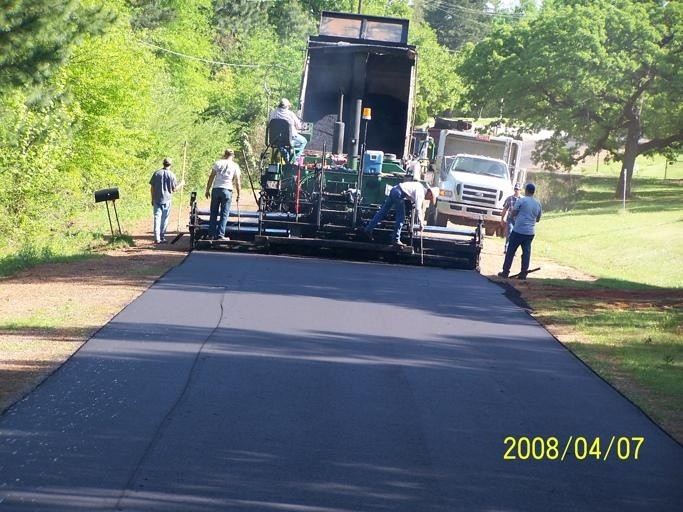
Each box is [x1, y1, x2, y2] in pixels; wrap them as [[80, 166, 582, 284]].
[[426, 182, 440, 205], [163, 158, 170, 165], [223, 149, 234, 157], [513, 183, 520, 189], [280, 98, 290, 106], [526, 183, 534, 191]]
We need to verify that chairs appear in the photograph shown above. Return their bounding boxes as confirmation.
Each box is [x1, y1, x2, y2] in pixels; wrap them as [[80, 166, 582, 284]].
[[264, 117, 295, 164]]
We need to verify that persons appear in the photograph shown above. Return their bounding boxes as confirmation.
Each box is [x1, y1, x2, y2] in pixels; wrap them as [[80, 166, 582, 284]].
[[361, 181, 440, 246], [500, 184, 523, 256], [269, 97, 307, 165], [204, 148, 242, 241], [147, 157, 186, 245], [497, 183, 542, 280]]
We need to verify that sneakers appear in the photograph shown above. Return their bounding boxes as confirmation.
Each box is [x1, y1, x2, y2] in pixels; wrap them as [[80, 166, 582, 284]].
[[205, 235, 215, 239], [392, 239, 406, 247], [515, 275, 525, 280], [219, 236, 230, 240], [498, 272, 508, 278]]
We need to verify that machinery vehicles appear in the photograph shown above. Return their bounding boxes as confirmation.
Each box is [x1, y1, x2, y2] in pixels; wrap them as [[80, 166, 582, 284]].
[[186, 9, 483, 269]]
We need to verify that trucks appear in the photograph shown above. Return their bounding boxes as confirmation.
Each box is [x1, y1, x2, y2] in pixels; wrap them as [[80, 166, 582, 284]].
[[426, 116, 526, 238]]
